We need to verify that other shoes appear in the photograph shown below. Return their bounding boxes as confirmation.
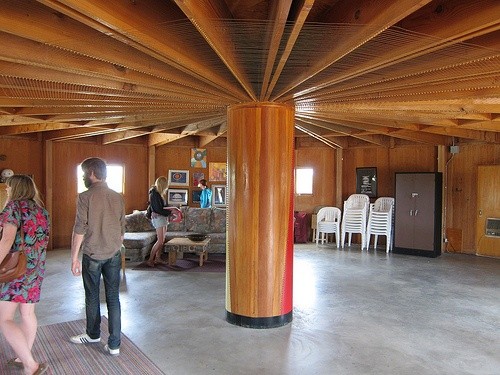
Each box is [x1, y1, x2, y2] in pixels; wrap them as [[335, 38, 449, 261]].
[[147, 260, 154, 267], [154, 256, 169, 265], [33, 363, 49, 375], [7, 358, 24, 369]]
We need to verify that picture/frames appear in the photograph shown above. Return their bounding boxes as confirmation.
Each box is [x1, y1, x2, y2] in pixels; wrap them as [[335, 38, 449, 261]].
[[167, 188, 189, 205], [356, 167, 378, 197], [167, 170, 190, 186], [193, 173, 204, 187], [209, 162, 228, 182], [211, 185, 227, 207]]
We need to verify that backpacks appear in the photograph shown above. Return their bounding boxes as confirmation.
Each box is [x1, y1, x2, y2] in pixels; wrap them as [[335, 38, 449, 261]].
[[146, 205, 152, 222]]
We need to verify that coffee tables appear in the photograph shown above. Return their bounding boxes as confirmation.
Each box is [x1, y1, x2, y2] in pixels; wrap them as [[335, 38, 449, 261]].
[[165, 238, 211, 268]]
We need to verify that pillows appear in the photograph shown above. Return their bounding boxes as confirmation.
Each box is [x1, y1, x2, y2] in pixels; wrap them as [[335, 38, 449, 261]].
[[124, 209, 229, 234]]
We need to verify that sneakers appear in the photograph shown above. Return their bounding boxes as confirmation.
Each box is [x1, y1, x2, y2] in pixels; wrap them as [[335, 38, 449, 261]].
[[69, 333, 101, 343], [104, 343, 120, 355]]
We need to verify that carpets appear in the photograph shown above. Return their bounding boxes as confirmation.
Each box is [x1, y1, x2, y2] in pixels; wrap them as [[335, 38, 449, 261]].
[[0, 315, 166, 375]]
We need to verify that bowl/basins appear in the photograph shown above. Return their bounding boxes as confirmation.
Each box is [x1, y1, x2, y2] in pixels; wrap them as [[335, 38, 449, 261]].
[[185, 234, 211, 241]]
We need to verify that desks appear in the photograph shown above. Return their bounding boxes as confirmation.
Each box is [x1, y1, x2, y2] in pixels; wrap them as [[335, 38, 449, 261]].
[[312, 205, 339, 242]]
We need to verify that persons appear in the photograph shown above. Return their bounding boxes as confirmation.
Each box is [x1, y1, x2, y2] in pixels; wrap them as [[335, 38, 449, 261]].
[[200, 180, 212, 209], [0, 174, 50, 375], [70, 156, 127, 357], [145, 176, 176, 268]]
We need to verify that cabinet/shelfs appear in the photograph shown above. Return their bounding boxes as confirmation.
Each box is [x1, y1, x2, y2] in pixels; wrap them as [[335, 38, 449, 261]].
[[394, 172, 444, 257]]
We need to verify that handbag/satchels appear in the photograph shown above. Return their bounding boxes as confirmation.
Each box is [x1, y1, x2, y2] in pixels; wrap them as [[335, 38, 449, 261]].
[[0, 251, 27, 282]]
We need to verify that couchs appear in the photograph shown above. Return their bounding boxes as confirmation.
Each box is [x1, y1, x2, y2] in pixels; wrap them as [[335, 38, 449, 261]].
[[294, 211, 308, 242], [122, 208, 233, 262]]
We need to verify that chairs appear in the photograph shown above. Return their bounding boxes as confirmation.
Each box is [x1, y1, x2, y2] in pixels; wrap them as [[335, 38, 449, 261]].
[[316, 194, 395, 253]]
